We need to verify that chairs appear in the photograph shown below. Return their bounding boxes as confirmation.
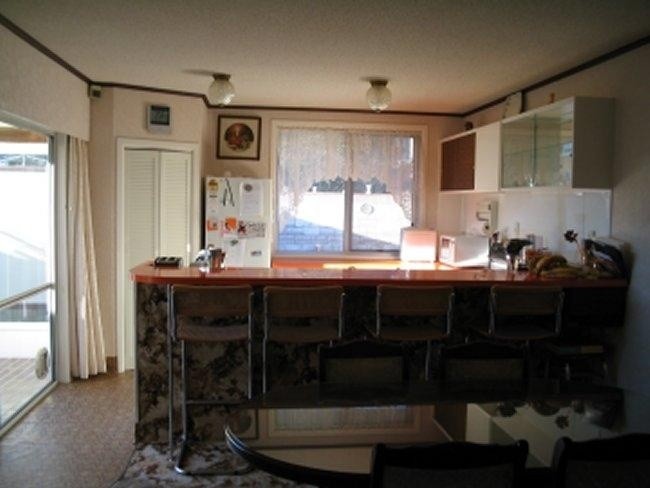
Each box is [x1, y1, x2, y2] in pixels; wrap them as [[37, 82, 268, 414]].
[[551, 432, 650, 488], [167, 282, 257, 475], [369, 438, 529, 488]]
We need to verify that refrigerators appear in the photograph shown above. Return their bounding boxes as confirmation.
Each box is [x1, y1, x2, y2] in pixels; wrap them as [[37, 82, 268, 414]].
[[204, 176, 275, 269]]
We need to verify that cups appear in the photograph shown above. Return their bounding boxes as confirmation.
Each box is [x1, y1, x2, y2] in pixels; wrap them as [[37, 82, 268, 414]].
[[209, 248, 222, 274]]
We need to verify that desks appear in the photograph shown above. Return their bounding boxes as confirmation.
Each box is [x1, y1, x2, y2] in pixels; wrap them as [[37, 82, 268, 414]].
[[221, 376, 650, 488]]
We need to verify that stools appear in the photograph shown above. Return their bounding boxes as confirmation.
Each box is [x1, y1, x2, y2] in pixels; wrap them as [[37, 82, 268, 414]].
[[468, 286, 570, 396], [262, 284, 351, 396], [364, 284, 456, 396]]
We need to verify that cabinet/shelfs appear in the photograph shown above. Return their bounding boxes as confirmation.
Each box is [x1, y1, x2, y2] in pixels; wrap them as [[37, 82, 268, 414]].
[[475, 122, 502, 192], [500, 97, 612, 195], [438, 129, 475, 194]]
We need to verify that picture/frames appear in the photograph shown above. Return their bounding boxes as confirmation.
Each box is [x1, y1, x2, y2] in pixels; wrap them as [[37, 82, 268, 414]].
[[215, 114, 262, 161]]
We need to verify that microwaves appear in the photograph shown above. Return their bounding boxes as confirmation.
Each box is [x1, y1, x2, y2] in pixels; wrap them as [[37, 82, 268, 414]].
[[439, 234, 490, 269]]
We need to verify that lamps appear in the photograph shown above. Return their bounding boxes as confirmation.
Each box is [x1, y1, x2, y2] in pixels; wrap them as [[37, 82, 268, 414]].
[[365, 78, 392, 113], [206, 73, 235, 106]]
